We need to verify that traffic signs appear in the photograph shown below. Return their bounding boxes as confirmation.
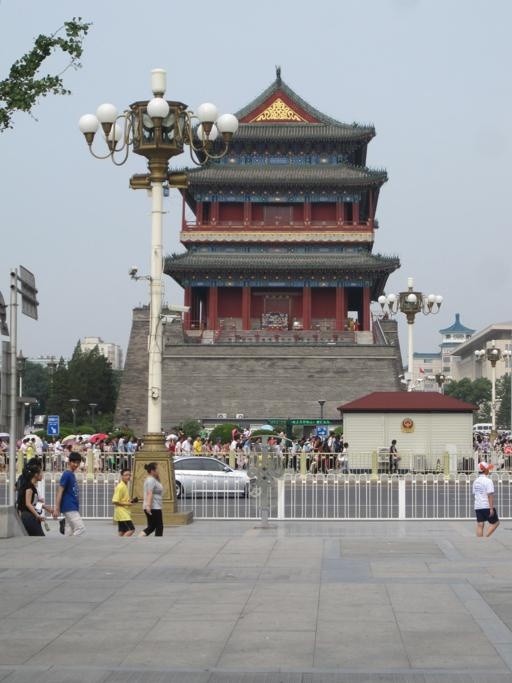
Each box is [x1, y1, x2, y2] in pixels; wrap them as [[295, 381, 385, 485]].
[[48, 415, 60, 436]]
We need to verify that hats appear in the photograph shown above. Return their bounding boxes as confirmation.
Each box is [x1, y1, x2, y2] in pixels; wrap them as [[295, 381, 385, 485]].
[[477, 462, 493, 473]]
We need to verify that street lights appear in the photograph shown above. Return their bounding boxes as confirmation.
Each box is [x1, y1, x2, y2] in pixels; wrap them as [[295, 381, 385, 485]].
[[376, 277, 445, 391], [426, 373, 453, 394], [474, 338, 512, 431], [77, 68, 239, 529]]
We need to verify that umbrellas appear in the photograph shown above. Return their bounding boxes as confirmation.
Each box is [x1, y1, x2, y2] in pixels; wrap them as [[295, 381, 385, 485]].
[[60, 433, 110, 444]]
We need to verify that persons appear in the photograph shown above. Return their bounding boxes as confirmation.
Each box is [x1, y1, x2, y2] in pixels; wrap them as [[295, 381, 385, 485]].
[[161, 424, 350, 474], [473, 461, 500, 538], [390, 440, 402, 472], [0, 434, 144, 536], [472, 428, 512, 470], [138, 462, 163, 537]]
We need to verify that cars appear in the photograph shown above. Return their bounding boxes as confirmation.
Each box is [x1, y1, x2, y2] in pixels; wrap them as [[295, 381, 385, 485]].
[[224, 434, 295, 456], [173, 455, 250, 499]]
